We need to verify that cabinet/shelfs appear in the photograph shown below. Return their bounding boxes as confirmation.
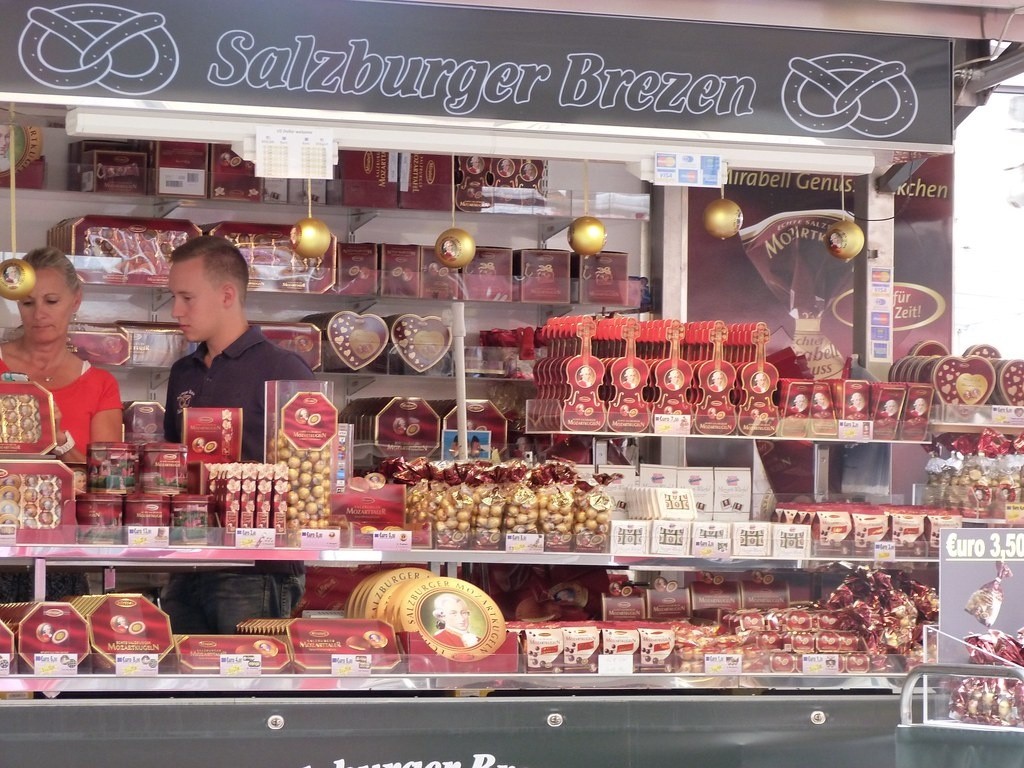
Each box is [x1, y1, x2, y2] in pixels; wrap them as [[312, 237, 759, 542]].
[[0, 93, 1024, 694], [895, 663, 1024, 768]]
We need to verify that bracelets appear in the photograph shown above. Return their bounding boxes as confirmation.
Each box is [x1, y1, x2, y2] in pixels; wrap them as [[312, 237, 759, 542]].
[[51, 430, 76, 456]]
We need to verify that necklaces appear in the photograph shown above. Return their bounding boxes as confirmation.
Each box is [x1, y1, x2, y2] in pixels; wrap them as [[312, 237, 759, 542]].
[[24, 350, 68, 382]]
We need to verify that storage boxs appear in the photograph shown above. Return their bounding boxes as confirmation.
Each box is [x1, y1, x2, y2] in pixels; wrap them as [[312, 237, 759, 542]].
[[68, 136, 549, 209], [531, 316, 1024, 561], [47, 215, 630, 305], [248, 310, 453, 374], [1, 381, 510, 547], [0, 155, 46, 188], [0, 568, 873, 677], [63, 320, 195, 366]]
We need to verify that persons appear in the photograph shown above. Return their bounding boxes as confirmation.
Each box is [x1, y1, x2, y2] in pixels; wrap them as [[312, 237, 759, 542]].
[[162, 237, 319, 636], [1, 248, 123, 604]]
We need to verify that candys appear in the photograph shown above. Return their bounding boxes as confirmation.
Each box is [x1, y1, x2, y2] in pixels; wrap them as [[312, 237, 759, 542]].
[[0, 392, 64, 532], [266, 427, 609, 553], [921, 451, 1024, 518]]
